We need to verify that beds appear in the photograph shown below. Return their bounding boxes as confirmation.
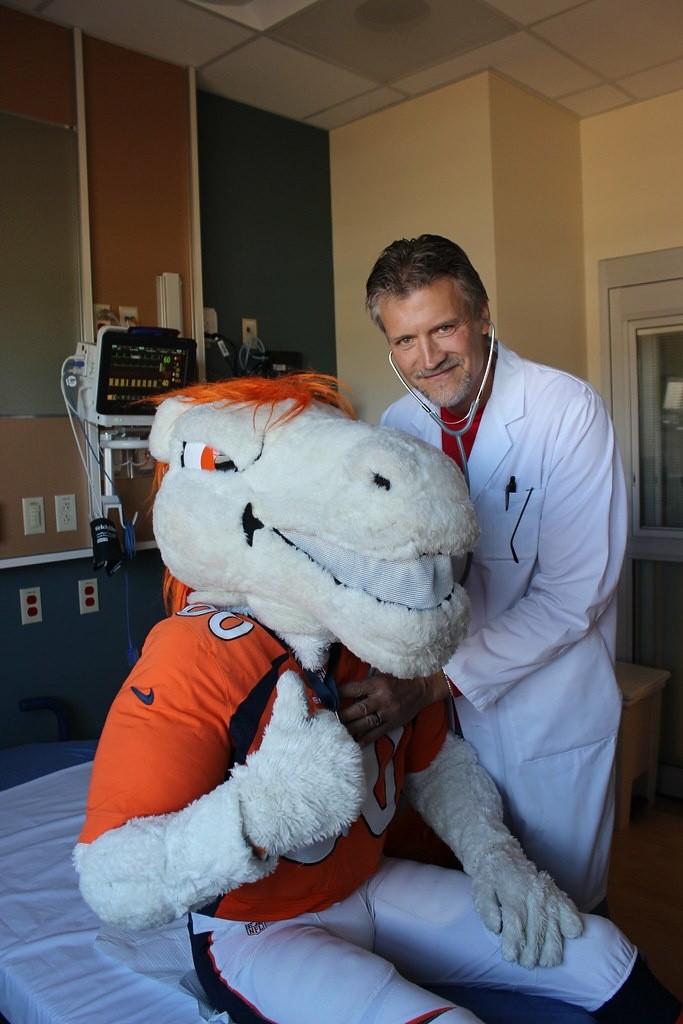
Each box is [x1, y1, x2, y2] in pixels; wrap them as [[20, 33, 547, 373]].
[[0, 756, 233, 1024]]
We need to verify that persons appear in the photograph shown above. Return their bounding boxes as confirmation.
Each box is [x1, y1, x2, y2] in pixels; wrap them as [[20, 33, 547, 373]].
[[362, 236, 631, 919]]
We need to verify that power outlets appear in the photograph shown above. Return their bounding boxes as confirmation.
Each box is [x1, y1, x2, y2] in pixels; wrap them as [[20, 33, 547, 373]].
[[118, 306, 138, 329], [78, 577, 100, 614], [242, 318, 258, 351], [92, 304, 112, 341], [20, 586, 42, 625], [55, 492, 77, 532], [202, 307, 218, 350]]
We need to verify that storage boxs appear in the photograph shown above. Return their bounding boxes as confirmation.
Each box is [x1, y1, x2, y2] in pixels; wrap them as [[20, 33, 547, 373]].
[[613, 659, 671, 829]]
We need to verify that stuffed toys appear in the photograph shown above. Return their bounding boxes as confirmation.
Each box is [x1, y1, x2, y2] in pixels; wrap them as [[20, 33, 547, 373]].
[[70, 371, 585, 970]]
